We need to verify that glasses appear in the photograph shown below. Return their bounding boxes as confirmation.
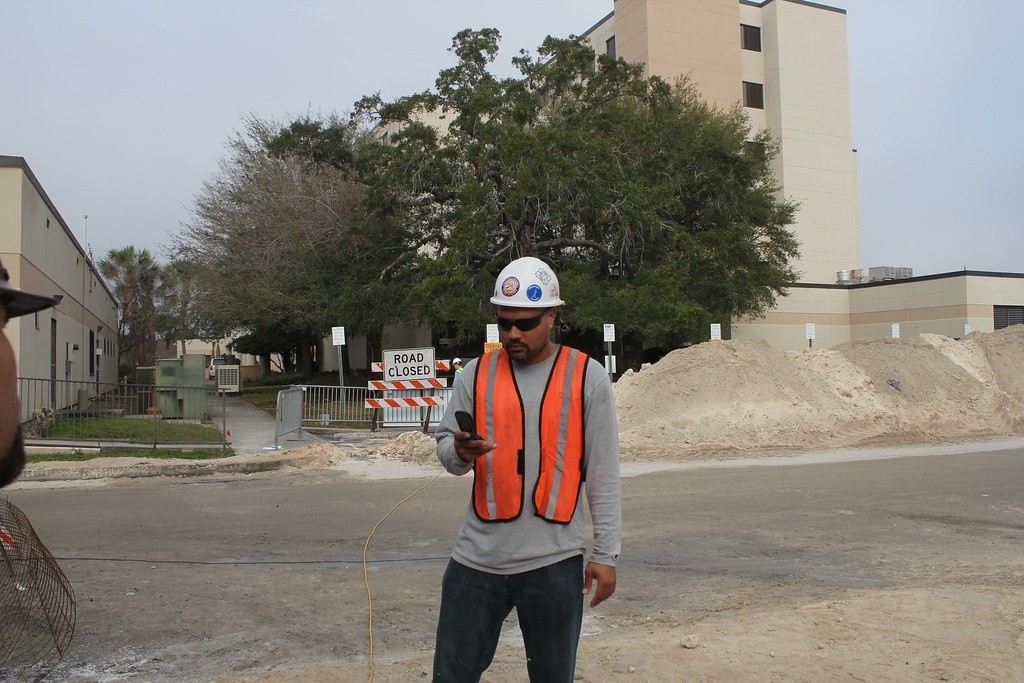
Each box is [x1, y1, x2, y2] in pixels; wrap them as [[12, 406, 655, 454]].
[[496, 307, 549, 332]]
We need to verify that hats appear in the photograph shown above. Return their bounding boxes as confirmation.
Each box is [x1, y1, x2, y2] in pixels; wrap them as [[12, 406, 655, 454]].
[[0, 258, 59, 318]]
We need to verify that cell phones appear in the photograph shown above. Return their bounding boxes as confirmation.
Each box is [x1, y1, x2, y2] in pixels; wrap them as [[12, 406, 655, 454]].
[[454, 411, 486, 449]]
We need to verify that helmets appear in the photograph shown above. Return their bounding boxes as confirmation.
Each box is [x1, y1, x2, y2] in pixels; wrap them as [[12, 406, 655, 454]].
[[453, 358, 461, 365], [490, 258, 565, 308]]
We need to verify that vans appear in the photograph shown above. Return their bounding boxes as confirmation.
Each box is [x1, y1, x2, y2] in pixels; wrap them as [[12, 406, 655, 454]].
[[206, 357, 226, 380]]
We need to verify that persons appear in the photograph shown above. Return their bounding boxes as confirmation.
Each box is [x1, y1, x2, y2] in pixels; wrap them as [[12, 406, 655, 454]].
[[432, 257, 622, 683], [0, 260, 60, 502], [452, 357, 464, 387]]
